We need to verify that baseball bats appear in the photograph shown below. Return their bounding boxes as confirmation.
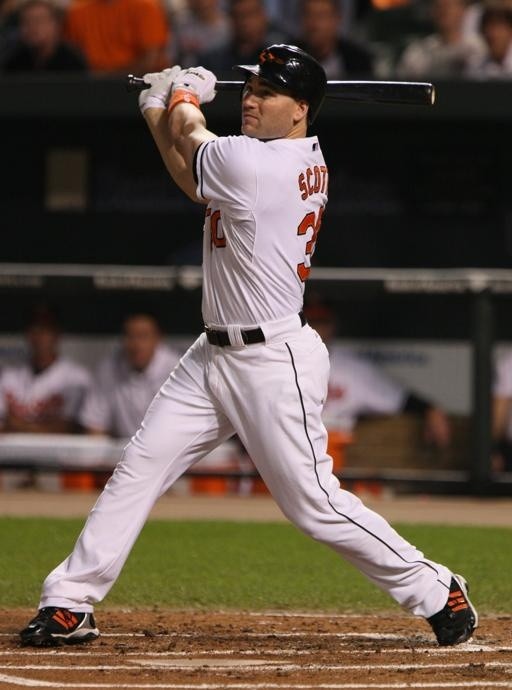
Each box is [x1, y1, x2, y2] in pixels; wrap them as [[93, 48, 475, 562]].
[[128, 75, 435, 105]]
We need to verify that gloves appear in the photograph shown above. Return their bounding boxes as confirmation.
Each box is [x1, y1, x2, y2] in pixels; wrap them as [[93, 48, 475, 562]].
[[170, 66, 217, 104], [138, 64, 181, 114]]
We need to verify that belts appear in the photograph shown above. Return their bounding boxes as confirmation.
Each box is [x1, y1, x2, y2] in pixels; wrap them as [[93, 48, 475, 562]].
[[204, 311, 310, 346]]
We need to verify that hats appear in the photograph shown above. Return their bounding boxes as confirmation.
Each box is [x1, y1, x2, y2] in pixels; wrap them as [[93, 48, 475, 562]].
[[232, 43, 328, 126]]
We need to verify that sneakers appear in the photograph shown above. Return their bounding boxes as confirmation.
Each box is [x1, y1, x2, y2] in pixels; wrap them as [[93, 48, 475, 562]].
[[427, 574, 480, 648], [20, 606, 101, 646]]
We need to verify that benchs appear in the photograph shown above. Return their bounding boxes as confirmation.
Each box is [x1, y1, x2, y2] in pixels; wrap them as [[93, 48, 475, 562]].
[[345, 411, 510, 498]]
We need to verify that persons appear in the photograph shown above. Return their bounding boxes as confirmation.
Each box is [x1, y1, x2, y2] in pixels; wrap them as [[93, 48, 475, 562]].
[[0, 313, 92, 491], [488, 340, 512, 475], [0, 0, 512, 89], [69, 307, 227, 497], [305, 299, 453, 474], [17, 41, 482, 646]]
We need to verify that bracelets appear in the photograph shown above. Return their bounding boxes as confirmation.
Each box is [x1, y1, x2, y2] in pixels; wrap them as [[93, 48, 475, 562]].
[[403, 392, 433, 417], [169, 89, 200, 113], [140, 96, 167, 115], [488, 432, 504, 458]]
